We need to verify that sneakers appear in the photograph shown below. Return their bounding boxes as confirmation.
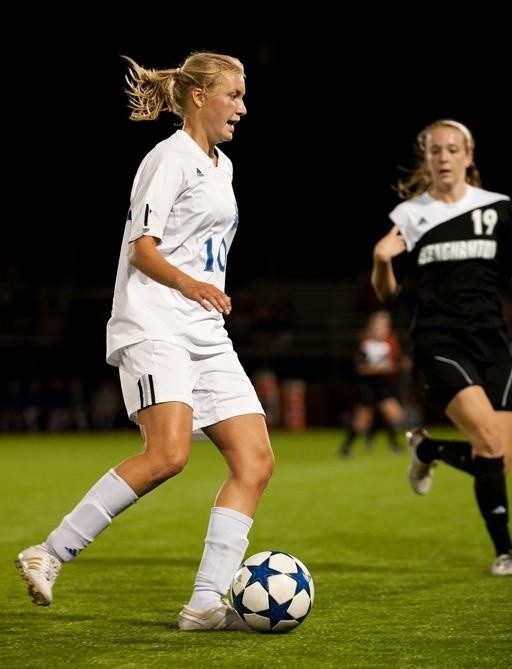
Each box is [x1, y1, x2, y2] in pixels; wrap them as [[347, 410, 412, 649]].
[[14, 546, 62, 606], [178, 599, 251, 631], [492, 554, 512, 575], [406, 427, 433, 495]]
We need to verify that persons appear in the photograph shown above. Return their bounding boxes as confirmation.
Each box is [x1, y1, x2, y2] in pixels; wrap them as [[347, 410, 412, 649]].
[[14, 49, 277, 631], [370, 118, 512, 575], [336, 311, 405, 460], [18, 319, 89, 431], [255, 327, 304, 430]]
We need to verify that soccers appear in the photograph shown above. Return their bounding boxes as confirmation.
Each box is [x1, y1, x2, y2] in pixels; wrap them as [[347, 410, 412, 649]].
[[231, 551, 314, 632]]
